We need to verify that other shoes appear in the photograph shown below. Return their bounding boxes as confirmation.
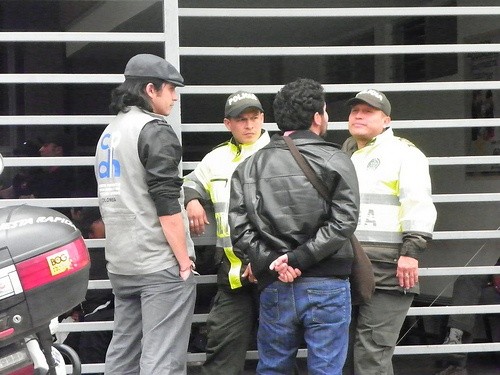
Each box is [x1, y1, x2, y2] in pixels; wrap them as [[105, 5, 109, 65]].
[[435, 364, 468, 375], [430, 352, 467, 361]]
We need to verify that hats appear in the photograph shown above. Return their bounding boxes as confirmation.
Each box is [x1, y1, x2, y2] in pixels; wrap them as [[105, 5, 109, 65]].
[[124, 54, 185, 87], [38, 129, 75, 150], [345, 89, 392, 116], [225, 91, 264, 119]]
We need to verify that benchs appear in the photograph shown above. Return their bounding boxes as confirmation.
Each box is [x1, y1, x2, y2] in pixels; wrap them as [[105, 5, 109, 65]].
[[396, 294, 500, 346]]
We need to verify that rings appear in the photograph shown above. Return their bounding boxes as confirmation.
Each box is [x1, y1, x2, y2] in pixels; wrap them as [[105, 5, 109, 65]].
[[495, 285, 498, 288], [189, 221, 194, 223]]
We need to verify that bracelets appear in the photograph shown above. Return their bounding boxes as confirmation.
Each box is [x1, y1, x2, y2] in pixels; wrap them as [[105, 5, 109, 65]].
[[180, 266, 192, 272]]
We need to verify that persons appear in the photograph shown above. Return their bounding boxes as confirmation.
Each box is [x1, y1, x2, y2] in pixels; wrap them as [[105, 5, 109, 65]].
[[94, 53, 198, 375], [0, 92, 500, 375], [228, 78, 362, 375]]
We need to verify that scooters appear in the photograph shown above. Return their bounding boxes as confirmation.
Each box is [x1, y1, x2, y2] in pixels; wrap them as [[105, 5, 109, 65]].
[[0, 203, 90, 374]]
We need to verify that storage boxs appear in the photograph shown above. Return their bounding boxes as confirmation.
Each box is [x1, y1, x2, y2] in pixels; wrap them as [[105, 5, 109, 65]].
[[0, 204, 91, 347]]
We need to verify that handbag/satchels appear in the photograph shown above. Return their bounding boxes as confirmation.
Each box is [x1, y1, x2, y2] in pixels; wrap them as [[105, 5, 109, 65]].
[[349, 233, 376, 304]]
